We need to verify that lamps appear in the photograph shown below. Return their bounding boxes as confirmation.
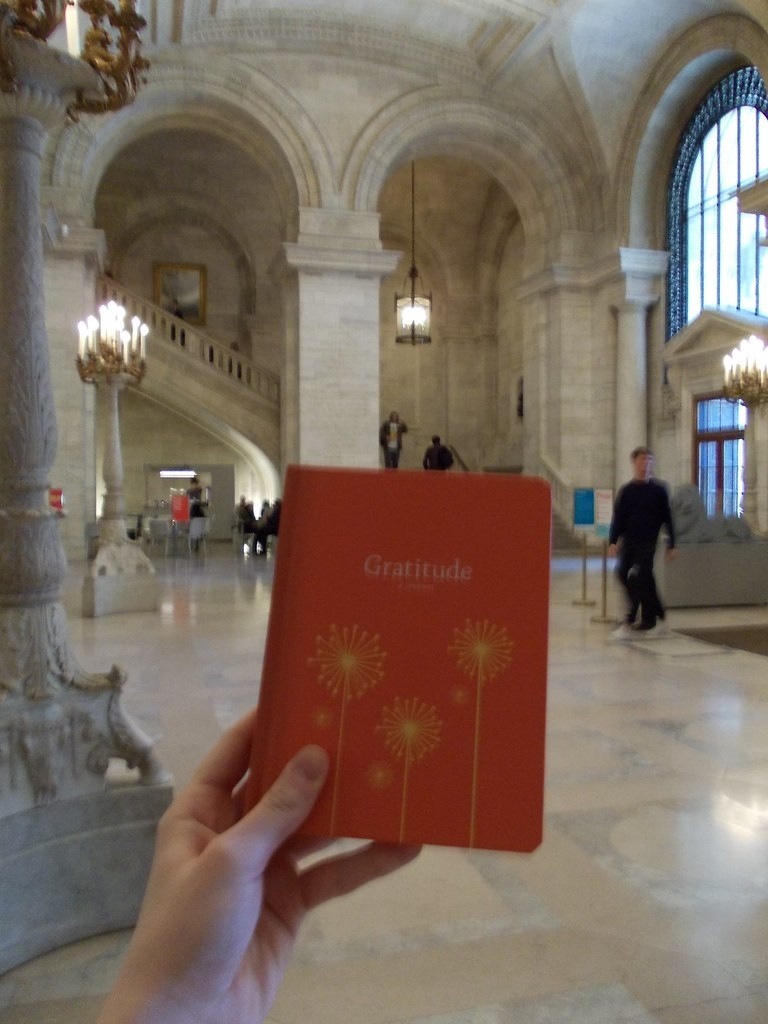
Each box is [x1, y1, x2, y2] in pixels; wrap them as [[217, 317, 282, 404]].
[[76, 300, 147, 536], [395, 158, 433, 347], [721, 336, 768, 526]]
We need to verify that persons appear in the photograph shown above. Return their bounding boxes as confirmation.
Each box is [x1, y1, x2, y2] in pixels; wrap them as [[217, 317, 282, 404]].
[[171, 299, 185, 344], [105, 262, 118, 280], [95, 709, 422, 1024], [235, 496, 281, 554], [187, 479, 203, 500], [230, 341, 241, 377], [609, 446, 676, 637], [379, 411, 408, 468], [422, 435, 448, 471], [189, 502, 208, 551]]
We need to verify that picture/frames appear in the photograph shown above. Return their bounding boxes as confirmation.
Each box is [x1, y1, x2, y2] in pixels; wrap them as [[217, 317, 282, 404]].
[[154, 262, 206, 325]]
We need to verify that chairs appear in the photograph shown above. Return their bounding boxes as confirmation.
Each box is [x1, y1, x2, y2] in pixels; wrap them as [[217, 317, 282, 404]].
[[137, 517, 212, 560], [232, 518, 278, 558]]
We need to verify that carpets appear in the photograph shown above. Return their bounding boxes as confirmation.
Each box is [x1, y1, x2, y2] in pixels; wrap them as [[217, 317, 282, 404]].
[[670, 622, 768, 658]]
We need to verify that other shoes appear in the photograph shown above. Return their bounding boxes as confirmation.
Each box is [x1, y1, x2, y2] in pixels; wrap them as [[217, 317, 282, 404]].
[[646, 619, 668, 636], [612, 623, 631, 640]]
